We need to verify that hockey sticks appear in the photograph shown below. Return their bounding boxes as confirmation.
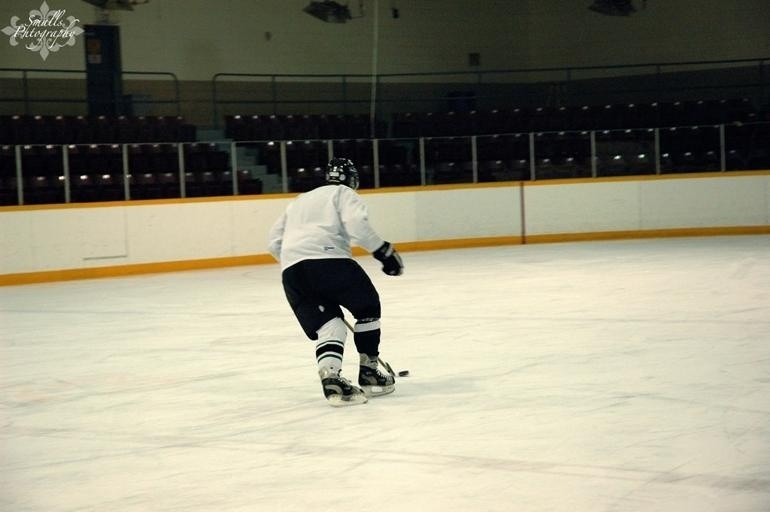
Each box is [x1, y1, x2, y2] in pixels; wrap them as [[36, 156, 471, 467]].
[[344, 318, 410, 376]]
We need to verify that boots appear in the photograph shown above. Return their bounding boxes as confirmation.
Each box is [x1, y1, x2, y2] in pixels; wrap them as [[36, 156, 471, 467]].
[[360, 364, 396, 388], [318, 367, 364, 399]]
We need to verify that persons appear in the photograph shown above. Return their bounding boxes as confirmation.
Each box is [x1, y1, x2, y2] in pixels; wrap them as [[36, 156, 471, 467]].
[[264, 155, 405, 403]]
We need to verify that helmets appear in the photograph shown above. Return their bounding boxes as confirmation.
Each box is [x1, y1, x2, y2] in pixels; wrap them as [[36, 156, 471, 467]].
[[325, 157, 360, 189]]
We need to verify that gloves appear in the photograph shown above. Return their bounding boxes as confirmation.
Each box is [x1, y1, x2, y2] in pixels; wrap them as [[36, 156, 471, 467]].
[[372, 241, 404, 277]]
